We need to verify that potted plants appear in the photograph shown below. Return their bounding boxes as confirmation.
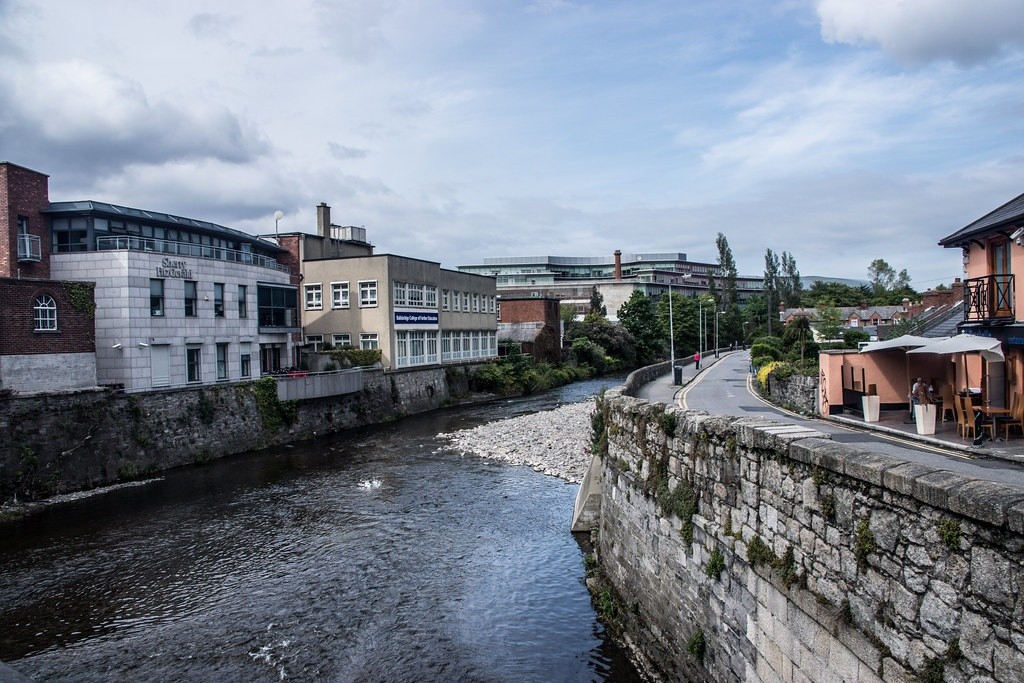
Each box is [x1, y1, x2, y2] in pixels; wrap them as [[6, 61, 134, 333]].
[[911, 383, 941, 434]]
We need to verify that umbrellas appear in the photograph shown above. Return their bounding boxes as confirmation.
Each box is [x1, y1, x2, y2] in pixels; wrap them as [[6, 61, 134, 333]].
[[859, 334, 1005, 396]]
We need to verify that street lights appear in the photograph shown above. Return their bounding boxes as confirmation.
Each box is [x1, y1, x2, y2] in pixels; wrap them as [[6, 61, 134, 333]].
[[698, 294, 714, 369], [742, 321, 750, 350], [716, 311, 727, 359], [668, 272, 692, 386]]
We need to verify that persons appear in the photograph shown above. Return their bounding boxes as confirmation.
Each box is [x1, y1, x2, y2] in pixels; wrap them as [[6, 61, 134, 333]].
[[913, 378, 928, 419], [694, 351, 700, 369]]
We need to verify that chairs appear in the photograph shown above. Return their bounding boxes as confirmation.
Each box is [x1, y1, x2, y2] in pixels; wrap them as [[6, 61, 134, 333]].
[[954, 392, 1024, 442], [943, 384, 958, 423]]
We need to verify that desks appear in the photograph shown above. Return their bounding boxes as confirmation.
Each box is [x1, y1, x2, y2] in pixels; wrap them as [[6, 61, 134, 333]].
[[959, 391, 981, 398], [972, 405, 1011, 441]]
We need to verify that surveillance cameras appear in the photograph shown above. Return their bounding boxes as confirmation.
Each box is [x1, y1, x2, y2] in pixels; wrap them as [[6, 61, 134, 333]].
[[1010, 226, 1024, 246]]
